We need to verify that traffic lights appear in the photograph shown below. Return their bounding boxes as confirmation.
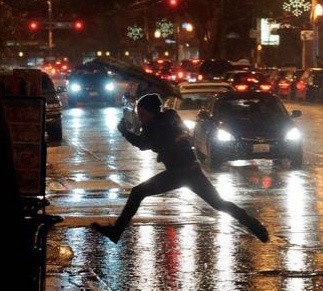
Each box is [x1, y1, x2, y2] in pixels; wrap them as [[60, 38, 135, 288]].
[[29, 20, 85, 32]]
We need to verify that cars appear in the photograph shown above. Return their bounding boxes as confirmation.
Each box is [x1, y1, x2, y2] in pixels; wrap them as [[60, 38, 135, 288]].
[[40, 58, 323, 138], [15, 68, 61, 145], [64, 63, 116, 108], [191, 91, 303, 170]]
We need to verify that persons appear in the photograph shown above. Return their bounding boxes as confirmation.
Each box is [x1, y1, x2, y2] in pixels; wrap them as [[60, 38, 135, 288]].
[[89, 93, 270, 244]]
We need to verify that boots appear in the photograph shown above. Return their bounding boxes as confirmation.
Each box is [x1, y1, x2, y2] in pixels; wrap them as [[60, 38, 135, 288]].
[[91, 216, 130, 244], [238, 209, 269, 243]]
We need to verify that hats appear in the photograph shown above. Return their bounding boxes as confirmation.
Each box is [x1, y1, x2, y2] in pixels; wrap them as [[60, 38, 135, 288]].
[[137, 93, 163, 115]]
